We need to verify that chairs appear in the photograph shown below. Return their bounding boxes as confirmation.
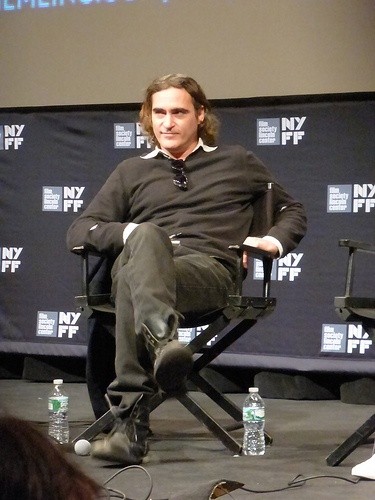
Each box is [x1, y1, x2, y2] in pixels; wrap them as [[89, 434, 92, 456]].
[[70, 179, 274, 455], [324, 238, 375, 466]]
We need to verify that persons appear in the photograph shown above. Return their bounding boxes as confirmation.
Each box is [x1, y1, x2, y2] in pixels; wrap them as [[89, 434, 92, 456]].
[[0, 413, 112, 500], [66, 74, 308, 465]]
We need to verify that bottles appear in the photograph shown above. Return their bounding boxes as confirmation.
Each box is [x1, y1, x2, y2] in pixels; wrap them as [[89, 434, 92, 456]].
[[47, 379, 69, 445], [242, 387, 266, 456]]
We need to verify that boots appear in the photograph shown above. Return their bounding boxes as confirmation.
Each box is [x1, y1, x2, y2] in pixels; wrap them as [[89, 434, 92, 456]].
[[140, 309, 189, 402], [90, 398, 148, 466]]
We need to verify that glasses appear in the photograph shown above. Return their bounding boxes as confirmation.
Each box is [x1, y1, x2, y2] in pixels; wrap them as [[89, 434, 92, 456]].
[[172, 158, 189, 191]]
